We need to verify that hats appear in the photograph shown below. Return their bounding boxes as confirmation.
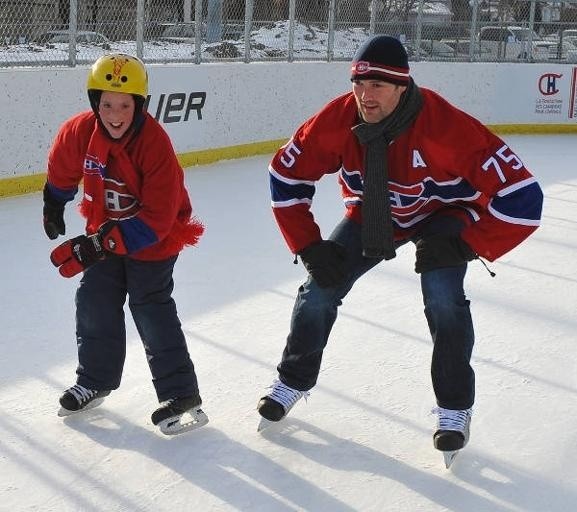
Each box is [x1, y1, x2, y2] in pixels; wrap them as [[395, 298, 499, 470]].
[[349, 36, 409, 87]]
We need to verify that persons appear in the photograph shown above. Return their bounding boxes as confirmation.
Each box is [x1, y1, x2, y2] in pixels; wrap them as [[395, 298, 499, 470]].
[[256, 33, 542, 450], [43, 53, 203, 424]]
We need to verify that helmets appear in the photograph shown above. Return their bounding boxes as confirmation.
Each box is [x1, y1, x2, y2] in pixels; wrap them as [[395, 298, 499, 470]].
[[86, 52, 148, 101]]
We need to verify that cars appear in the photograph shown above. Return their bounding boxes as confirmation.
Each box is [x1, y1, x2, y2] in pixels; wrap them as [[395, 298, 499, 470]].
[[416, 25, 577, 63], [157, 23, 258, 42], [32, 28, 112, 45]]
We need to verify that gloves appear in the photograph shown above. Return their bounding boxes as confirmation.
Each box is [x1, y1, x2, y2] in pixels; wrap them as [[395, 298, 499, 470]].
[[298, 239, 349, 289], [42, 181, 74, 239], [414, 230, 476, 273], [50, 221, 127, 278]]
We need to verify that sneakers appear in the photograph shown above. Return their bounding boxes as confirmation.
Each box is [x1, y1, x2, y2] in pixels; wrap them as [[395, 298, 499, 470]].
[[59, 384, 111, 411], [256, 375, 310, 421], [433, 404, 471, 451], [151, 393, 202, 425]]
